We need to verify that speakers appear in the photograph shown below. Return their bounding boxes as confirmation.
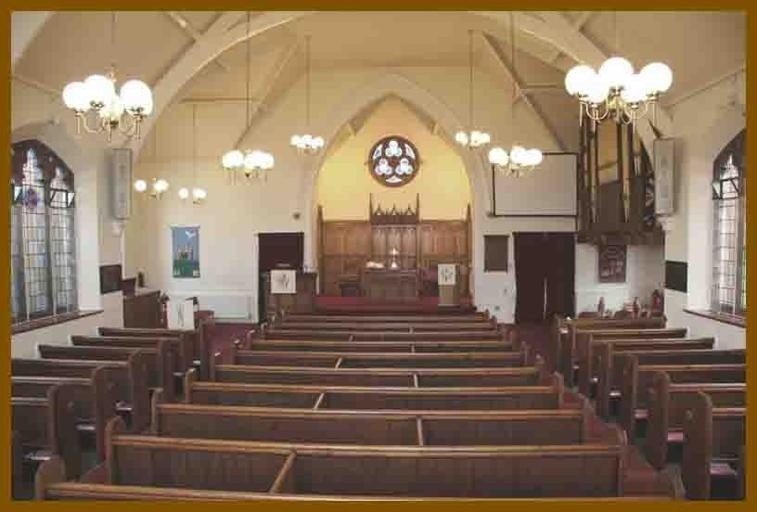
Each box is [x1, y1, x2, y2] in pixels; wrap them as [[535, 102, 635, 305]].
[[112, 148, 132, 218], [653, 138, 675, 216]]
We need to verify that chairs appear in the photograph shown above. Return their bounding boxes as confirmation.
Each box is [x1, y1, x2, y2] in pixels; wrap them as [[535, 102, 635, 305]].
[[185, 296, 215, 328]]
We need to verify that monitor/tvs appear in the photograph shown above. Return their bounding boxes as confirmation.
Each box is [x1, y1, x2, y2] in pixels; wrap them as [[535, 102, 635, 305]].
[[100, 265, 122, 293], [665, 261, 687, 293]]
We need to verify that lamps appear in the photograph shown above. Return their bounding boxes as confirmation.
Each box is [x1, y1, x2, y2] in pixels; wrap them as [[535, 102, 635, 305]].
[[62, 11, 153, 143], [290, 35, 325, 156], [455, 29, 491, 151], [221, 11, 274, 185], [488, 11, 543, 179], [564, 11, 674, 135], [177, 103, 207, 206], [134, 121, 169, 201]]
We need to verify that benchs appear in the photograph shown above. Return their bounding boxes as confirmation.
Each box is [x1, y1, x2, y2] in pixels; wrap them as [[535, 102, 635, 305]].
[[34, 296, 746, 500], [11, 323, 208, 502]]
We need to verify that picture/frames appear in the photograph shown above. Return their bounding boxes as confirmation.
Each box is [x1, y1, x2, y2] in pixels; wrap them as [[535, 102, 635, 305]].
[[652, 138, 675, 214], [113, 147, 133, 220], [100, 264, 122, 294], [665, 260, 687, 292]]
